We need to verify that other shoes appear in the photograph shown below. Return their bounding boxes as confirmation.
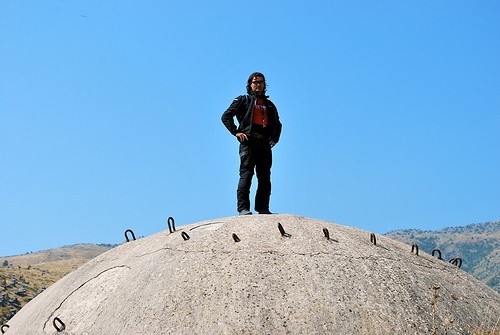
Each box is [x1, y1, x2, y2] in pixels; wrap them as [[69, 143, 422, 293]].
[[239, 209, 252, 215]]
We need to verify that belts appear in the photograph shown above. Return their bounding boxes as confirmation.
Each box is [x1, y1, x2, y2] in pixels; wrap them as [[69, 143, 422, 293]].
[[250, 133, 270, 139]]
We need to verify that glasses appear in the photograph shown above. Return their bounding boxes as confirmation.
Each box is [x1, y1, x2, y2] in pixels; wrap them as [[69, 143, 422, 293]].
[[251, 81, 265, 84]]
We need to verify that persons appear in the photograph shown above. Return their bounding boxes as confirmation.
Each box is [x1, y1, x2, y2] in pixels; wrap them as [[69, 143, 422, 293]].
[[220, 71, 282, 214]]
